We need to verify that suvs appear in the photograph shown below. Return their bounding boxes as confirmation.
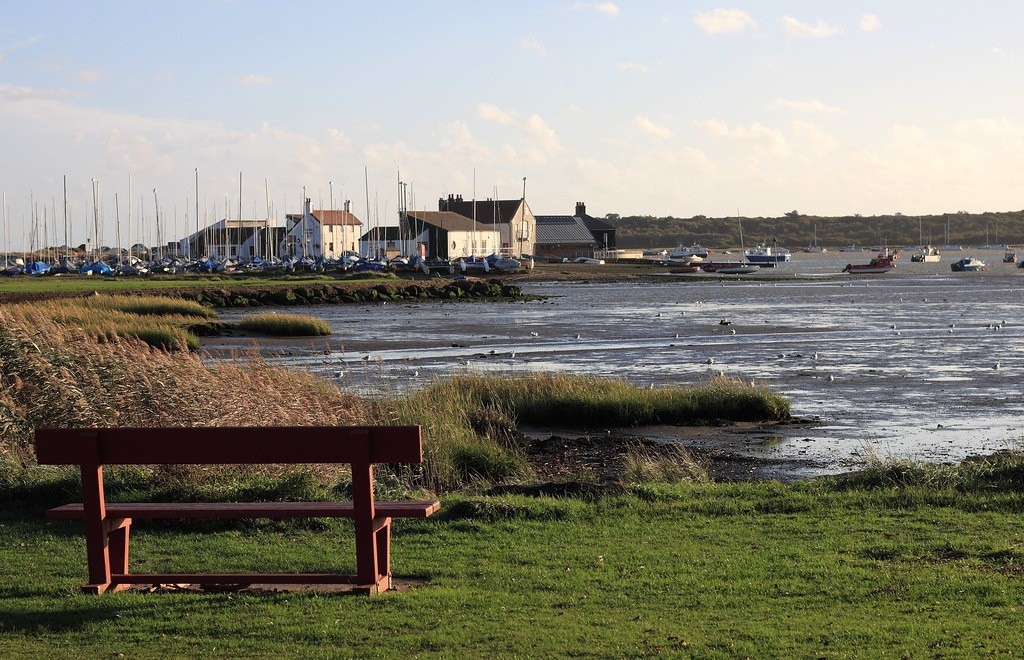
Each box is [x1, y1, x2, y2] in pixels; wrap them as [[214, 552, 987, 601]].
[[574, 256, 605, 264]]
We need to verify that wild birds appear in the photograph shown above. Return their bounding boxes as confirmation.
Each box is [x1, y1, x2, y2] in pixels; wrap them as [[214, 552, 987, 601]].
[[986, 324, 993, 329], [413, 371, 418, 377], [810, 351, 817, 360], [509, 351, 515, 358], [992, 363, 1000, 370], [950, 324, 956, 328], [922, 298, 927, 302], [899, 297, 903, 302], [890, 324, 896, 330], [531, 331, 538, 337], [894, 332, 901, 336], [996, 324, 1002, 332], [730, 329, 736, 334], [335, 370, 344, 378], [1002, 320, 1008, 324], [681, 311, 685, 315], [948, 328, 954, 334], [675, 333, 680, 339], [778, 354, 785, 358], [718, 319, 731, 327], [824, 374, 834, 382], [656, 313, 662, 317], [362, 355, 370, 360], [703, 358, 713, 364], [575, 334, 580, 340]]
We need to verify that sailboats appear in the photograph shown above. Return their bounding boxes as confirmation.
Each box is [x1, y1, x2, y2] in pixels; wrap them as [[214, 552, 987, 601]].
[[1, 164, 527, 277], [802, 223, 827, 254]]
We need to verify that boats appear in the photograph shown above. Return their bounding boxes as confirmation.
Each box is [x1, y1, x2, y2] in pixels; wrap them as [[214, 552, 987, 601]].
[[702, 260, 760, 273], [950, 256, 986, 271], [843, 246, 900, 274], [670, 241, 709, 258], [1003, 248, 1017, 262], [745, 241, 792, 263], [669, 266, 701, 273], [911, 243, 941, 263]]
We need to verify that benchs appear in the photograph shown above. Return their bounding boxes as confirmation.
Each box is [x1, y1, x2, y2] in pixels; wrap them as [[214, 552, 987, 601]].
[[33, 426, 441, 600]]
